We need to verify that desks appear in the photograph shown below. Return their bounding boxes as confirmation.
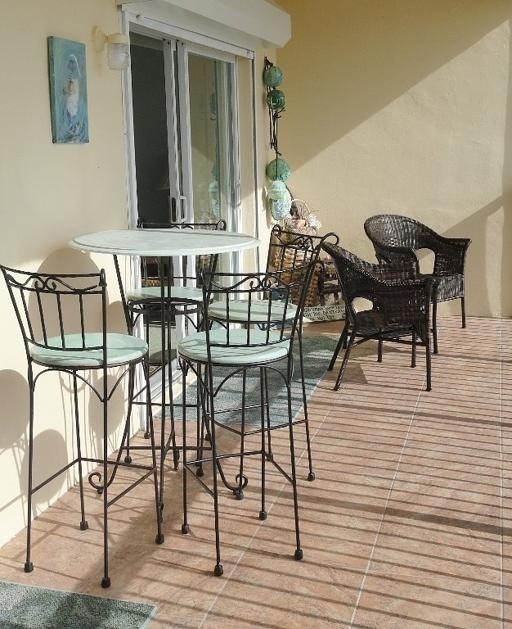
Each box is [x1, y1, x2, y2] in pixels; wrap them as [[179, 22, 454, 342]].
[[65, 230, 261, 499]]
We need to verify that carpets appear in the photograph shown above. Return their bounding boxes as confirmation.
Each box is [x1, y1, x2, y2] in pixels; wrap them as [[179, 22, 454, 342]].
[[0, 578, 158, 629]]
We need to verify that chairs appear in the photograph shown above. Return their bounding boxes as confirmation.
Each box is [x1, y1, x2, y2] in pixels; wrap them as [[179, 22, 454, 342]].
[[363, 213, 472, 354], [197, 224, 341, 493], [176, 259, 320, 576], [2, 265, 165, 589], [121, 219, 226, 464], [320, 241, 437, 392]]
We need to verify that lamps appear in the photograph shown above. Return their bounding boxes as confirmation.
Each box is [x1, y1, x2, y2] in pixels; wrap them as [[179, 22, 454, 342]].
[[92, 25, 130, 71]]
